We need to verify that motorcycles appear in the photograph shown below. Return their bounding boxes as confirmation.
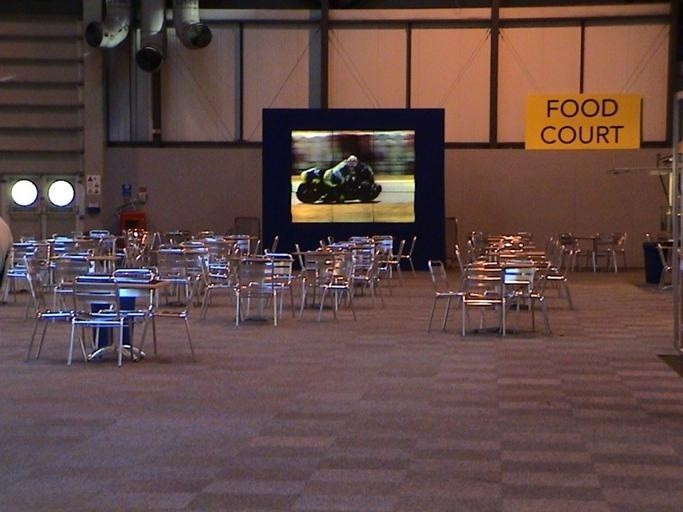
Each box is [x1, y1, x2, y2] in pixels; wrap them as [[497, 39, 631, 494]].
[[295, 165, 382, 203]]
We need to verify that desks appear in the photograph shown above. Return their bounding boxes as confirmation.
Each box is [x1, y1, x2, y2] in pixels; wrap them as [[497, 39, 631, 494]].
[[645, 233, 672, 291]]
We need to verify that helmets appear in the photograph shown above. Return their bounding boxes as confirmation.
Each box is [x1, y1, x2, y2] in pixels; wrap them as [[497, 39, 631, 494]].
[[347, 156, 358, 167]]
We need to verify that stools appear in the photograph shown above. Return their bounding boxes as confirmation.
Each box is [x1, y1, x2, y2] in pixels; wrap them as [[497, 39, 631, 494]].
[[119, 211, 146, 232]]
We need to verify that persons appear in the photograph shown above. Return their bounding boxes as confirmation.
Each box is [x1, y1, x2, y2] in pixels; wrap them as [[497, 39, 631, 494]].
[[323, 155, 358, 204]]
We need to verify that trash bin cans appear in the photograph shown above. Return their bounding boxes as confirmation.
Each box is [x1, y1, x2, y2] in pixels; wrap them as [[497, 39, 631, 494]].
[[643, 242, 668, 284]]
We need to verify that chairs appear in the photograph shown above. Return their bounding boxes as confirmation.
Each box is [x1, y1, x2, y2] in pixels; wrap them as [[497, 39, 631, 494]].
[[427, 227, 625, 337]]
